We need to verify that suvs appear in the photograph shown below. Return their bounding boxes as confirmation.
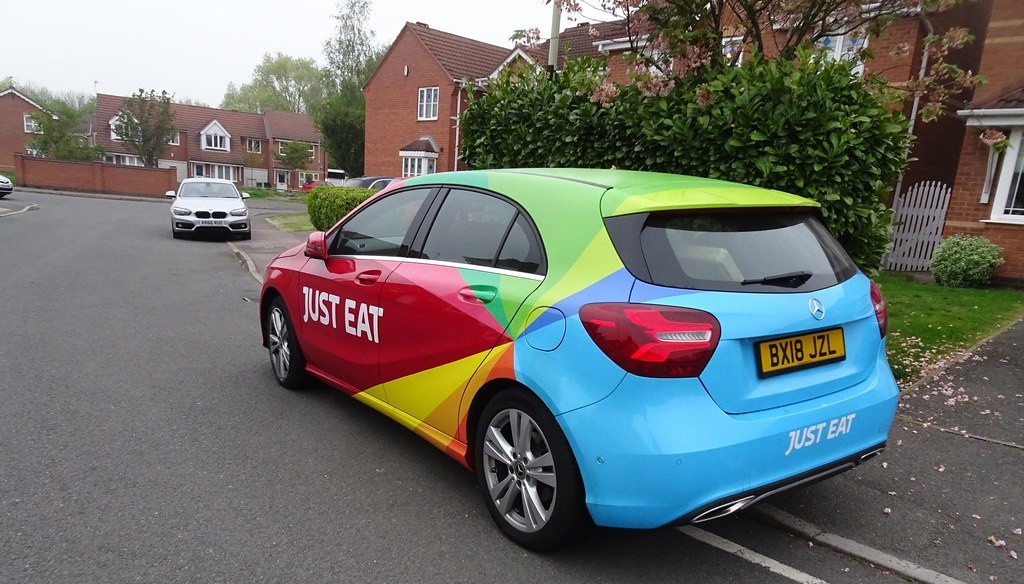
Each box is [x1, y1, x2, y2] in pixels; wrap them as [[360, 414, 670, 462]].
[[326, 169, 349, 186]]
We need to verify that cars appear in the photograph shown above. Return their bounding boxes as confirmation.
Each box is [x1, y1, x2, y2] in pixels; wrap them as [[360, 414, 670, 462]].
[[165, 177, 251, 241], [260, 166, 901, 554], [0, 174, 14, 199], [303, 180, 335, 193], [344, 175, 395, 193]]
